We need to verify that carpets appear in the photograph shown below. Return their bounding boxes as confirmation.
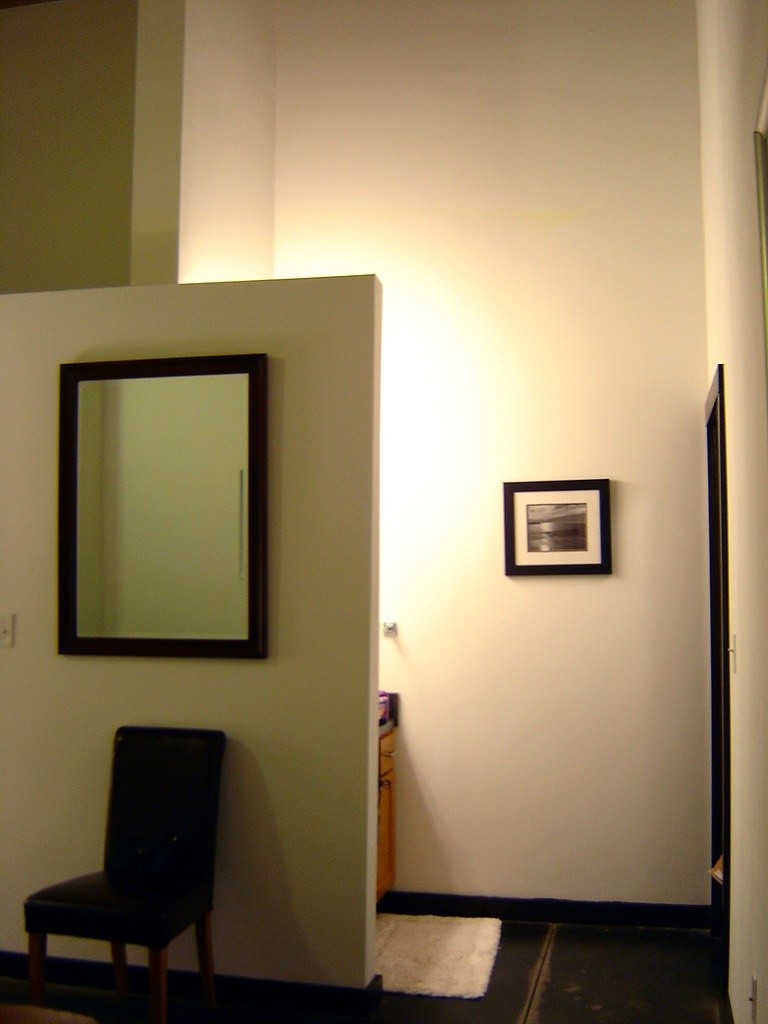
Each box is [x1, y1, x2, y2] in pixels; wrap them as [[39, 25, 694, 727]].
[[374, 913, 502, 999]]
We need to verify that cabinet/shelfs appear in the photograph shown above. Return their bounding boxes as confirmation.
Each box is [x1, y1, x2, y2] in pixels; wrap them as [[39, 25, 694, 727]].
[[376, 724, 399, 901]]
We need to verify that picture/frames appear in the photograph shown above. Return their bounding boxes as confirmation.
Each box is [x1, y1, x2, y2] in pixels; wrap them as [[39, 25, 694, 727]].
[[503, 477, 613, 577]]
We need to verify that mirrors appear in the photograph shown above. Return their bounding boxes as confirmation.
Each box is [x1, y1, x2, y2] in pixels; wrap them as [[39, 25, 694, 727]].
[[55, 351, 270, 659]]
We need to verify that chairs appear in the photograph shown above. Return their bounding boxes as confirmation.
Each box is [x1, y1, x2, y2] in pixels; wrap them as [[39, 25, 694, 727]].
[[23, 725, 226, 1023]]
